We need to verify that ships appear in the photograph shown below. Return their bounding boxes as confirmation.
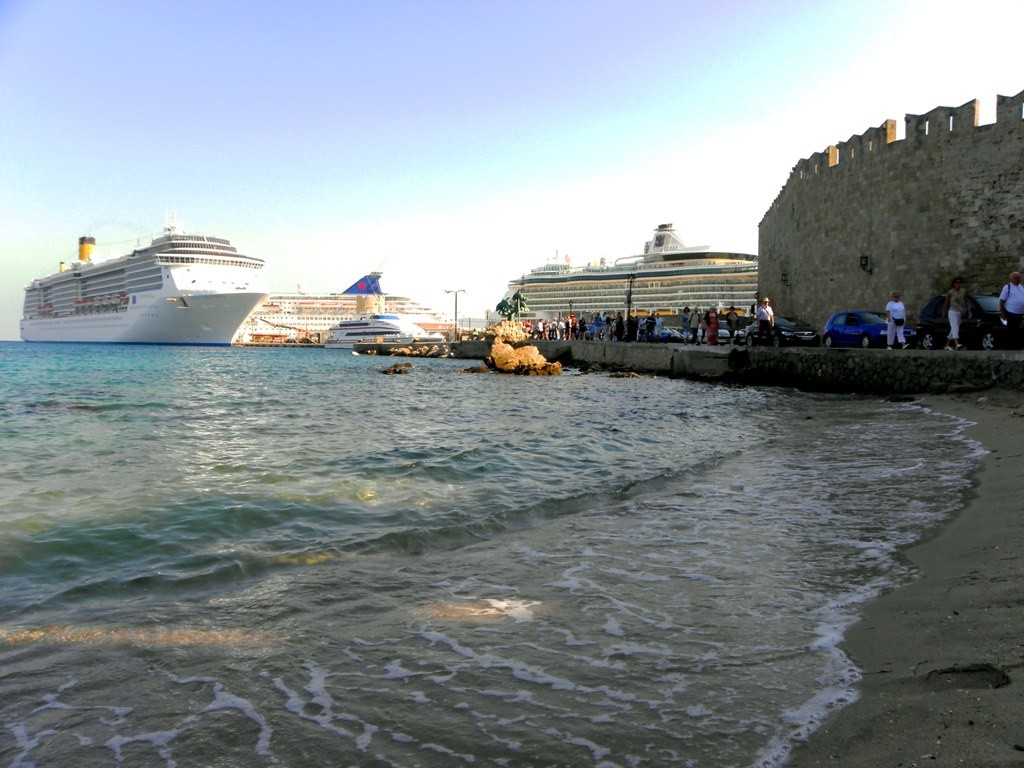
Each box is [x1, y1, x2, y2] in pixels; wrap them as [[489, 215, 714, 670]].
[[498, 222, 757, 328], [233, 290, 460, 346], [20, 218, 268, 348]]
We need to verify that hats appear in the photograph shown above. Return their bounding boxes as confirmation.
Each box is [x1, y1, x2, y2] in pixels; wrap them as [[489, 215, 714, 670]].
[[762, 297, 770, 302]]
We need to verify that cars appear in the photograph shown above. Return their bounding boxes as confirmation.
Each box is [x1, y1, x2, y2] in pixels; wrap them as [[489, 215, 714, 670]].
[[824, 310, 919, 349], [744, 314, 817, 348], [662, 326, 694, 343], [696, 320, 744, 345]]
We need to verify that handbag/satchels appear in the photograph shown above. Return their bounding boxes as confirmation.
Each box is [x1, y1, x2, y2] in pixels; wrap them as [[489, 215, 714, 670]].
[[961, 309, 970, 320], [895, 318, 905, 327]]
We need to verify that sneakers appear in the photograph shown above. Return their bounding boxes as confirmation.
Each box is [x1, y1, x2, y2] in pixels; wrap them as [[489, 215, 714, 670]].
[[944, 346, 954, 350], [902, 344, 909, 349], [955, 344, 963, 350], [887, 347, 894, 350]]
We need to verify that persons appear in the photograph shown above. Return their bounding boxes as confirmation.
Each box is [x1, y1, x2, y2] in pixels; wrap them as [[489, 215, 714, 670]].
[[470, 307, 707, 346], [725, 305, 740, 347], [756, 296, 775, 346], [884, 290, 911, 350], [999, 272, 1024, 349], [703, 305, 719, 346], [941, 276, 972, 351]]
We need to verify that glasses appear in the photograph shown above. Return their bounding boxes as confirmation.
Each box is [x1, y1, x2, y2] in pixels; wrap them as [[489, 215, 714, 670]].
[[956, 281, 962, 284], [894, 295, 899, 297]]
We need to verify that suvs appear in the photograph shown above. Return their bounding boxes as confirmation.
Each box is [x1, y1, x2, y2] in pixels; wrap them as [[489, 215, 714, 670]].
[[916, 294, 1019, 350]]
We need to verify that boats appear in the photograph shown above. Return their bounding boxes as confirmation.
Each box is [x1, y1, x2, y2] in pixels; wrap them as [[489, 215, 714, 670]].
[[323, 313, 447, 348]]
